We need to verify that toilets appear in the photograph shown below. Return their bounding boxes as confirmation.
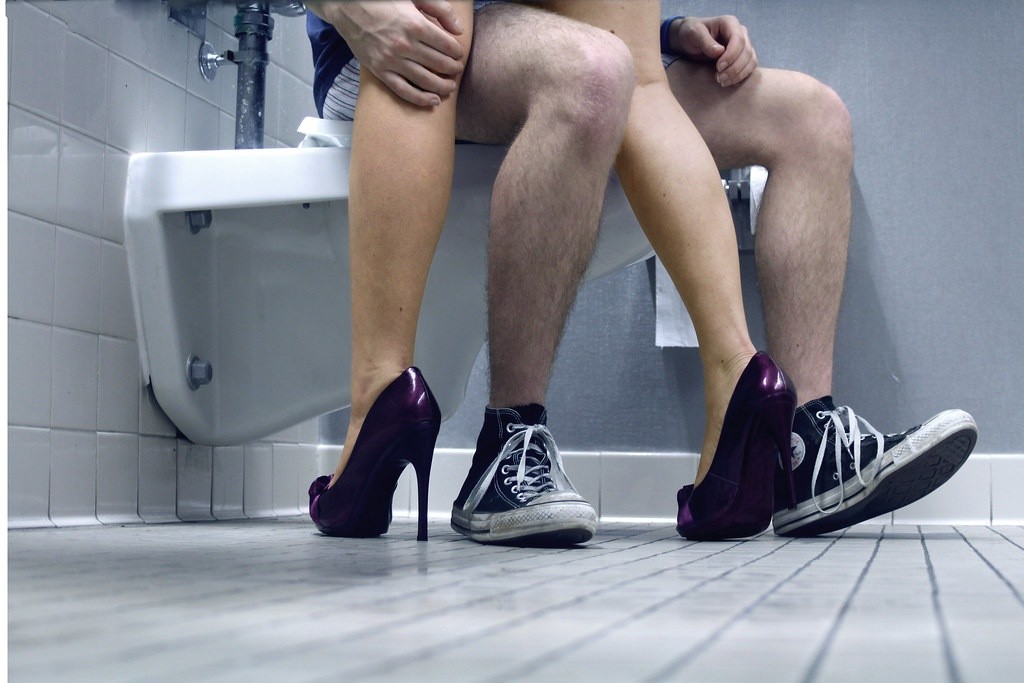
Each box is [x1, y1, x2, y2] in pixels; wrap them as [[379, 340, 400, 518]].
[[122, 132, 727, 447]]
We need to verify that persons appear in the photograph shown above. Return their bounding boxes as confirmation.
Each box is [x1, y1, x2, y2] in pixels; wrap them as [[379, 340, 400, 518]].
[[301, 0, 980, 548]]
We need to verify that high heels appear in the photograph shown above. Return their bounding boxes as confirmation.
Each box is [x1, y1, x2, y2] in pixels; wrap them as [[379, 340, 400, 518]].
[[308, 366, 443, 542], [675, 350, 800, 543]]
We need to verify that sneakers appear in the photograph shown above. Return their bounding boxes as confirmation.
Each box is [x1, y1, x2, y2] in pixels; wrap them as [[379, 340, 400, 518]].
[[449, 404, 598, 544], [771, 394, 979, 538]]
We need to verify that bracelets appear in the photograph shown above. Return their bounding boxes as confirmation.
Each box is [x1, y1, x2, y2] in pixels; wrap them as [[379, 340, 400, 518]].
[[661, 15, 685, 54]]
